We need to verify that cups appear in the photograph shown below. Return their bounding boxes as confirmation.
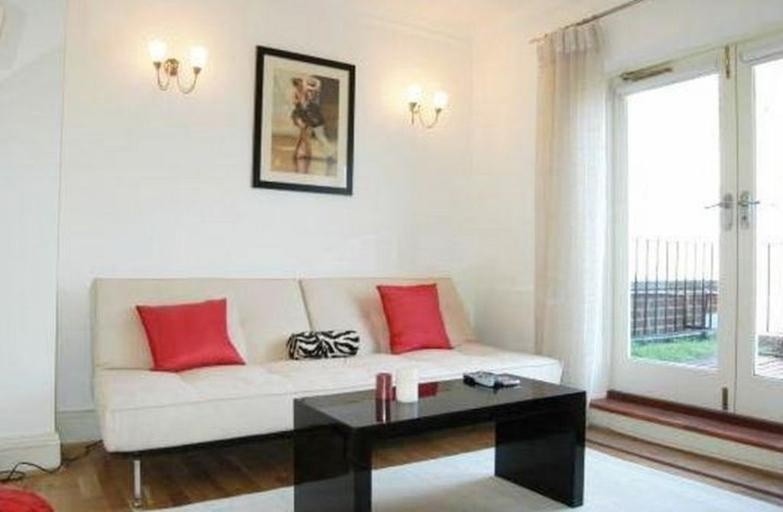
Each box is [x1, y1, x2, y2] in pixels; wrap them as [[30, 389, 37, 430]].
[[373, 373, 393, 401], [374, 401, 391, 422], [394, 403, 418, 423]]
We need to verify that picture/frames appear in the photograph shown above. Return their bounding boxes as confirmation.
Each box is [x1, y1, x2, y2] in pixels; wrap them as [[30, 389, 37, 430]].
[[249, 45, 357, 196]]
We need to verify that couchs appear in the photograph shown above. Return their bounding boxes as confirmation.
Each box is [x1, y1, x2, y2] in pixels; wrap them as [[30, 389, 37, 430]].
[[89, 275, 563, 509]]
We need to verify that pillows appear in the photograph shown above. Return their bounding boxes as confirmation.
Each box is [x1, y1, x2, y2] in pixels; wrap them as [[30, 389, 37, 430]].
[[285, 329, 361, 361], [374, 282, 452, 355], [129, 296, 247, 372]]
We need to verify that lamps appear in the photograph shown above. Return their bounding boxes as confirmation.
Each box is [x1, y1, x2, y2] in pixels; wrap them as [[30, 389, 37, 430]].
[[399, 82, 448, 130], [146, 37, 209, 95]]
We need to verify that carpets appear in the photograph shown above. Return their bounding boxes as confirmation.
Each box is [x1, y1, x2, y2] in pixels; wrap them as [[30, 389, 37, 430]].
[[150, 444, 783, 512]]
